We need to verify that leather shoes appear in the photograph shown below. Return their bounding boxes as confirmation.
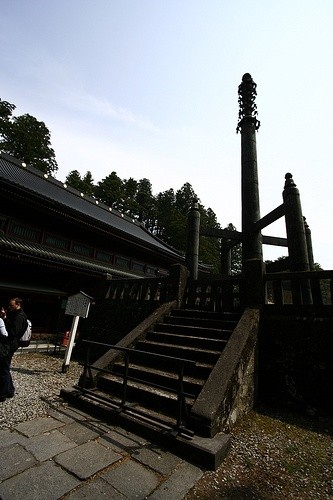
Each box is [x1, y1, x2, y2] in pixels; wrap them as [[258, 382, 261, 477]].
[[0, 390, 14, 402]]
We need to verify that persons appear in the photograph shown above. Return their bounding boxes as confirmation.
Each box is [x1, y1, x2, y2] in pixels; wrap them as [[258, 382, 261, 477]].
[[0, 297, 29, 403], [0, 306, 8, 337]]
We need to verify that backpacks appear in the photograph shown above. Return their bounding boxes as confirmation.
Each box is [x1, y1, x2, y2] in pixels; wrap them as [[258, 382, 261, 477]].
[[18, 319, 32, 347]]
